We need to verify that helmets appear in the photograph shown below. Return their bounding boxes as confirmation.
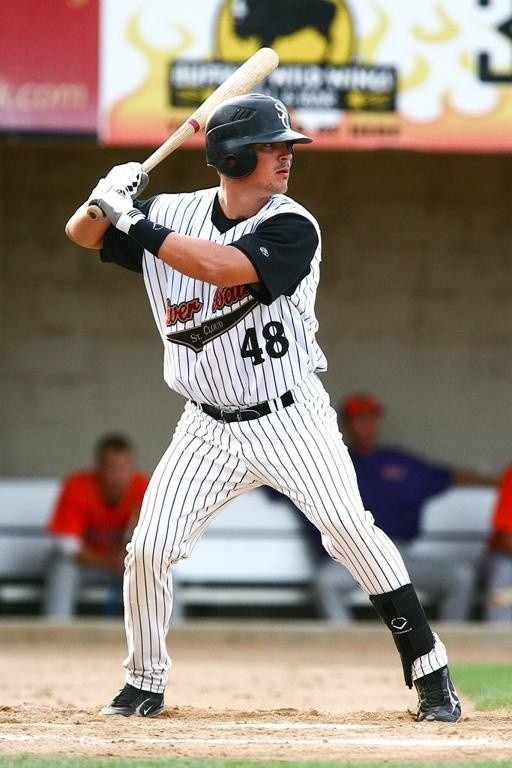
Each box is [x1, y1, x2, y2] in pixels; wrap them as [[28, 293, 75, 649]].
[[204, 94, 313, 180]]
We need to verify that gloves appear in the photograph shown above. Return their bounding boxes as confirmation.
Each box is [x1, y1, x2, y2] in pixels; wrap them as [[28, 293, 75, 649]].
[[88, 162, 147, 235]]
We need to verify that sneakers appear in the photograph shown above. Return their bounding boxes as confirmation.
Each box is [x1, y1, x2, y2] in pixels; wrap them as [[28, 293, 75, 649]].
[[96, 680, 169, 720], [412, 664, 460, 722]]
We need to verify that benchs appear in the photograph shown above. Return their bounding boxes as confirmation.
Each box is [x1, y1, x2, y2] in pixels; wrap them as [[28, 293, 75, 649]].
[[0, 470, 512, 617]]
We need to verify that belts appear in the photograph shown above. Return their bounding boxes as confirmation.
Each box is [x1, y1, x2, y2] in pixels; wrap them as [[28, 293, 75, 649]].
[[187, 387, 292, 421]]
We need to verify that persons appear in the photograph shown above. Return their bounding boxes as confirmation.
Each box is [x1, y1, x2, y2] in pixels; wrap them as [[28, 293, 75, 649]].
[[485, 461, 512, 622], [43, 434, 189, 622], [64, 94, 462, 724], [259, 394, 498, 622]]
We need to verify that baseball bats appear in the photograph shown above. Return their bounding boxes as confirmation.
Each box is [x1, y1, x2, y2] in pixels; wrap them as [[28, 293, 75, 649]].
[[86, 47, 280, 222]]
